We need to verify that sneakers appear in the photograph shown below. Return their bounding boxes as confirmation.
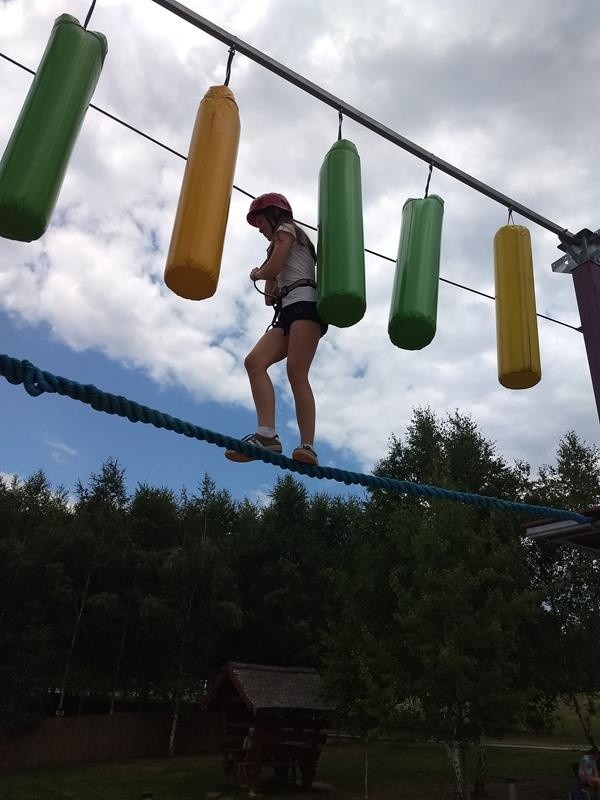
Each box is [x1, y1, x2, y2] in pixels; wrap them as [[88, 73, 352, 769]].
[[294, 445, 317, 465], [226, 432, 283, 462]]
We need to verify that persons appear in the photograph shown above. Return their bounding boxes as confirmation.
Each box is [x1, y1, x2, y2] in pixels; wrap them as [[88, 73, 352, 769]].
[[579, 744, 600, 789], [242, 728, 255, 749], [224, 195, 329, 467]]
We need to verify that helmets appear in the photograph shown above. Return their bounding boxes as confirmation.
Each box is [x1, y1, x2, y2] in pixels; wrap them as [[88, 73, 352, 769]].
[[247, 194, 292, 227]]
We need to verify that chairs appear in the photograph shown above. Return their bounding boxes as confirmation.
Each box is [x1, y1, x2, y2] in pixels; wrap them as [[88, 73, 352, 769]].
[[569, 762, 597, 800]]
